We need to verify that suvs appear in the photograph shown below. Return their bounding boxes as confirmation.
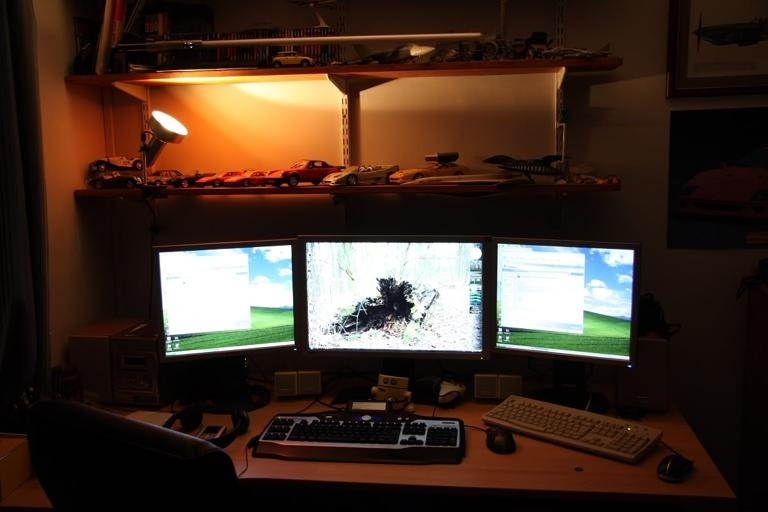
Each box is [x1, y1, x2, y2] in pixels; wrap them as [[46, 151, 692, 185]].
[[272, 51, 314, 67]]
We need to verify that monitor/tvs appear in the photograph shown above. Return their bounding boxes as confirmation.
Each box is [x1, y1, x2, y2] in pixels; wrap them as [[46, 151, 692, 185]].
[[493, 237, 641, 369], [152, 238, 299, 359], [297, 234, 492, 361]]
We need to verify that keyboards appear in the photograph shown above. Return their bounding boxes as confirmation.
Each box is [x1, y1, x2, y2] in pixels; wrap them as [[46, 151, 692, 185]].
[[252, 410, 465, 465], [481, 394, 663, 463]]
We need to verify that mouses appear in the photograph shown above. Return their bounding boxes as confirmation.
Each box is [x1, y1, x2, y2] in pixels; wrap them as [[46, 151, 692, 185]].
[[486, 425, 515, 455], [656, 454, 694, 484]]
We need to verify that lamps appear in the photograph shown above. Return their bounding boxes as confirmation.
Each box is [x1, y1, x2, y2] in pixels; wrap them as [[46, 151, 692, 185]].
[[142, 108, 189, 186]]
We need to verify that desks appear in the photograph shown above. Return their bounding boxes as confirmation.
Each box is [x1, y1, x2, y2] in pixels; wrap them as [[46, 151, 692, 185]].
[[0, 388, 738, 512]]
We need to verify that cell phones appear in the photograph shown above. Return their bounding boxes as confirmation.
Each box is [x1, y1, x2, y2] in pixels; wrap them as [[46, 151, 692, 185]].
[[197, 424, 227, 440]]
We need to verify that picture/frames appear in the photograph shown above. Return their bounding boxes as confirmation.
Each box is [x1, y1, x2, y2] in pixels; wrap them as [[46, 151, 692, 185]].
[[664, 105, 768, 249], [666, 0, 768, 99]]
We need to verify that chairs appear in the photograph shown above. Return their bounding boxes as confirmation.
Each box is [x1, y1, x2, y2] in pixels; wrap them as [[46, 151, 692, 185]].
[[27, 393, 249, 512]]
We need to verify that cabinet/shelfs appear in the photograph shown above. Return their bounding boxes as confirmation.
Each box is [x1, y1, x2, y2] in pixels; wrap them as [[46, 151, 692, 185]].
[[64, 49, 622, 201]]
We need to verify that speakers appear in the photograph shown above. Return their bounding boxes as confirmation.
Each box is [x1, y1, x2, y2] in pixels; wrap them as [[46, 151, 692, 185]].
[[63, 317, 145, 402], [473, 368, 522, 399], [617, 338, 672, 415], [274, 371, 322, 397]]
